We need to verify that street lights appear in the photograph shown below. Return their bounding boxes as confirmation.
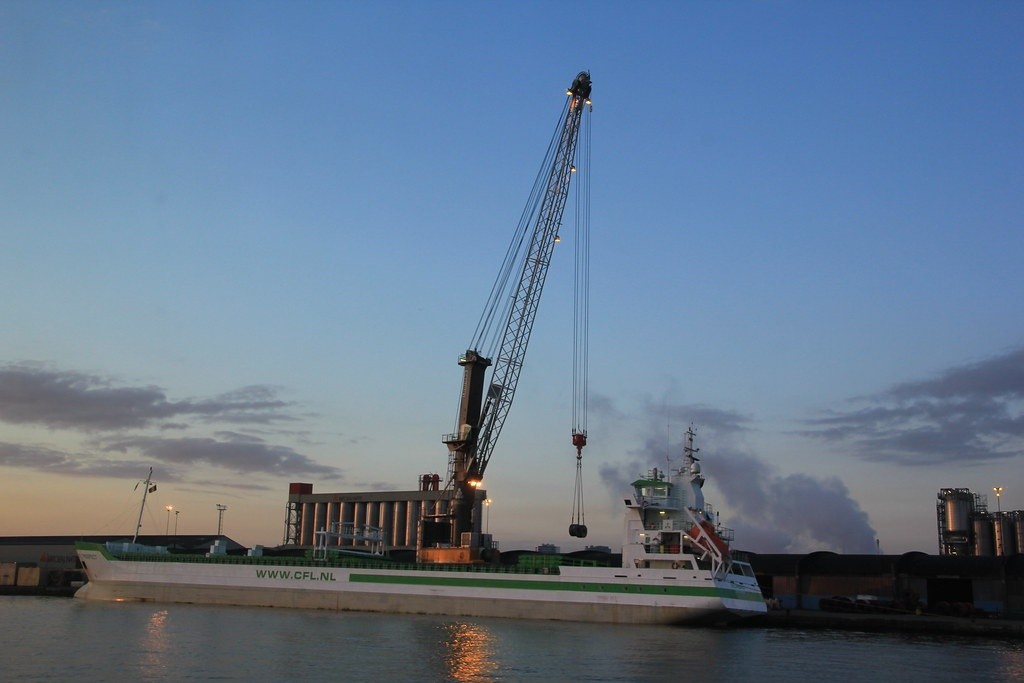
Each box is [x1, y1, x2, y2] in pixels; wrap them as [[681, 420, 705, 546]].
[[992, 486, 1006, 556], [175, 510, 182, 535], [165, 504, 172, 535]]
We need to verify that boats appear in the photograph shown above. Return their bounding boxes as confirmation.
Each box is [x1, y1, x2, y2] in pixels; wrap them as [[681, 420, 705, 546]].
[[75, 422, 771, 628]]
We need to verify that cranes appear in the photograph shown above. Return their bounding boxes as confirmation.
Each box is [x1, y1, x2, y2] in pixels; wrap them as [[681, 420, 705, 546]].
[[417, 67, 593, 561]]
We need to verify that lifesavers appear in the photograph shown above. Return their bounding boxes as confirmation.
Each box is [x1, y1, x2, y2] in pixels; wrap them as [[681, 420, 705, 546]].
[[671, 562, 679, 569], [652, 537, 660, 546]]
